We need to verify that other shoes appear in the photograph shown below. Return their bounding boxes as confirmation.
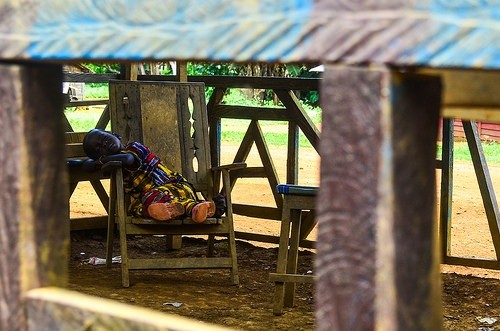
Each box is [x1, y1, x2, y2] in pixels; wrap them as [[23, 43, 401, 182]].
[[147, 202, 185, 221], [191, 200, 216, 223]]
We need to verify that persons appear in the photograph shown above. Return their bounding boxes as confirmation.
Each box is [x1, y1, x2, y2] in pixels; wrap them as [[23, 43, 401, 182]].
[[83, 129, 217, 224]]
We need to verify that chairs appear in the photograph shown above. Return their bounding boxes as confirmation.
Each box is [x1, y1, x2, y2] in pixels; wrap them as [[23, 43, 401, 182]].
[[102, 79, 246, 289], [268, 183, 322, 318]]
